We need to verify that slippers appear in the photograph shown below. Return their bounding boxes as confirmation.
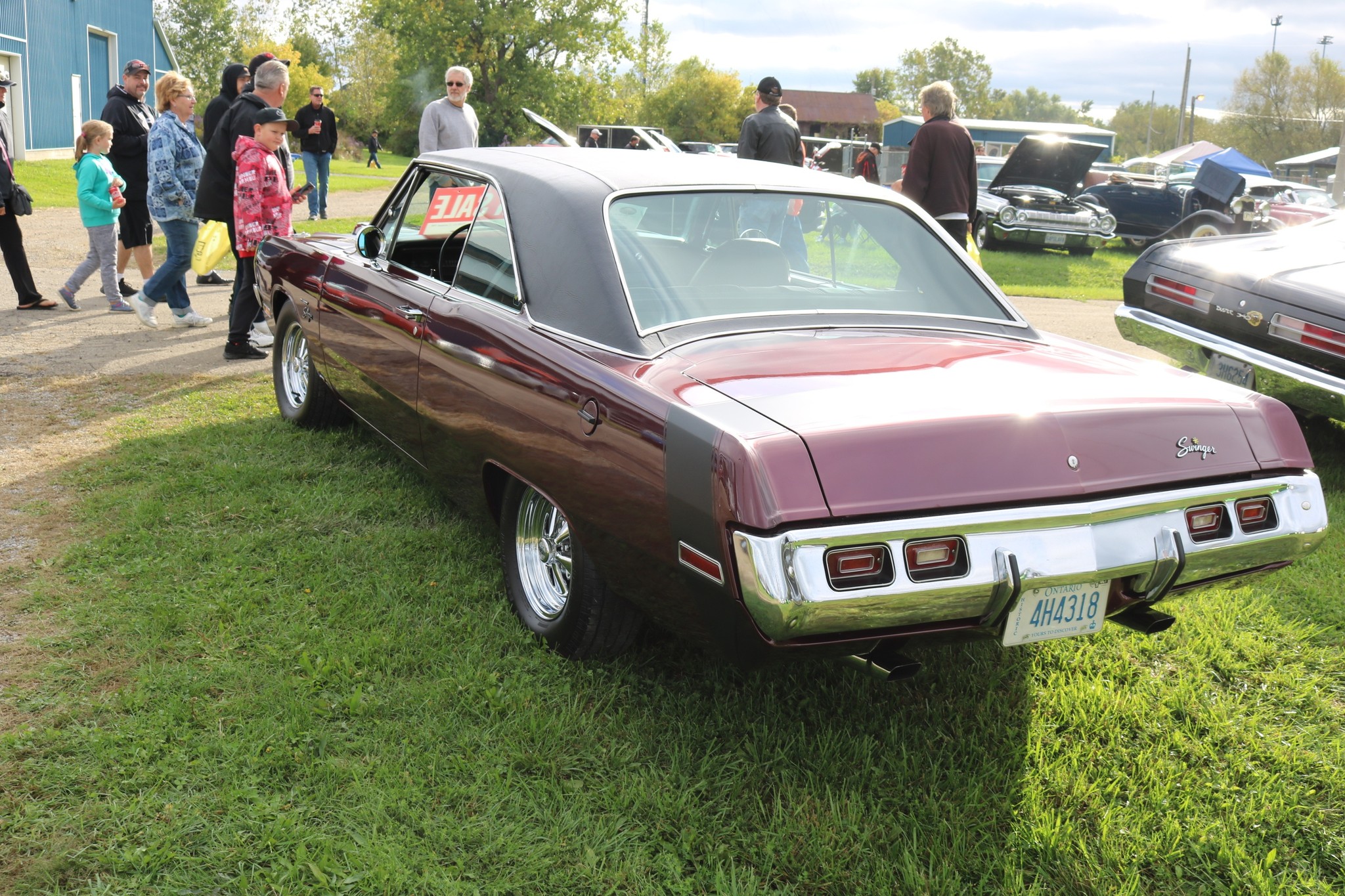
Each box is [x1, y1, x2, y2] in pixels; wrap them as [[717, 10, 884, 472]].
[[17, 299, 58, 310]]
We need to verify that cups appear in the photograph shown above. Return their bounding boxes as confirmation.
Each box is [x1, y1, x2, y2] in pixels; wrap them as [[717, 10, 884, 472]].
[[314, 119, 322, 133]]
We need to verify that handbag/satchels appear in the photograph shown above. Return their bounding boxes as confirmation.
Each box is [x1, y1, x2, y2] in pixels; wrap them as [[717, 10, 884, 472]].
[[11, 182, 34, 216], [190, 219, 231, 274]]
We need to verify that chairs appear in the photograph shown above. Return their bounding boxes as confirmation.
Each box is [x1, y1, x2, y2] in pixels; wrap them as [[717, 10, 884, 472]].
[[477, 253, 520, 315], [1275, 189, 1291, 204], [692, 232, 802, 286]]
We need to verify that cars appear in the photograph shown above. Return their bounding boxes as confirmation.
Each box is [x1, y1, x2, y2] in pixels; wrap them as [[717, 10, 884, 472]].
[[520, 109, 1337, 258], [252, 145, 1329, 665], [1112, 210, 1345, 424]]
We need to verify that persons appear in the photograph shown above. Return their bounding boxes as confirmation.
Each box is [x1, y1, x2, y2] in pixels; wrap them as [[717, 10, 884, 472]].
[[0, 72, 58, 310], [292, 86, 338, 220], [367, 129, 383, 168], [127, 71, 213, 329], [625, 135, 640, 150], [902, 81, 978, 254], [737, 77, 883, 273], [195, 52, 307, 358], [418, 66, 480, 208], [100, 59, 167, 302], [58, 120, 135, 314], [584, 129, 602, 148]]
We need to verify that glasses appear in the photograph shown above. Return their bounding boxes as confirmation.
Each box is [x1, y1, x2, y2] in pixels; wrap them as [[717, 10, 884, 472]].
[[446, 82, 466, 87], [597, 134, 599, 136], [124, 63, 150, 72], [313, 93, 324, 97]]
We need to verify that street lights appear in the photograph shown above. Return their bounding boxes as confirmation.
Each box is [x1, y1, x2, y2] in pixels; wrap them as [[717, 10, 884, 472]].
[[1316, 35, 1334, 117], [1270, 14, 1282, 52], [1189, 94, 1205, 144]]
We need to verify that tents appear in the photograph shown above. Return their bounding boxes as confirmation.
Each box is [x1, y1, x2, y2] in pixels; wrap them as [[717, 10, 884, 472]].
[[1145, 139, 1272, 178]]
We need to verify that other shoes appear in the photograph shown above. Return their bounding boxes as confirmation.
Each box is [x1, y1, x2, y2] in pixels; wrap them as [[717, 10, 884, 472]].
[[223, 340, 269, 359], [196, 269, 234, 285], [308, 215, 317, 220], [100, 277, 139, 297], [319, 209, 327, 219]]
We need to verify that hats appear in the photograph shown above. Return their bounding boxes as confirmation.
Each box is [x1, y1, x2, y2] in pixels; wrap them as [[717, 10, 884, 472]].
[[124, 59, 151, 77], [592, 129, 602, 136], [871, 141, 883, 154], [249, 52, 290, 76], [0, 74, 17, 87], [254, 107, 300, 131], [752, 77, 782, 97]]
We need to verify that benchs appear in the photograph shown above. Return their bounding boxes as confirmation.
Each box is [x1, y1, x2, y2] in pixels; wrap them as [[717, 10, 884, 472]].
[[635, 280, 932, 329]]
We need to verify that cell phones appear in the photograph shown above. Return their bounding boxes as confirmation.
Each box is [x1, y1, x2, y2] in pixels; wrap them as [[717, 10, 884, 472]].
[[291, 182, 315, 202]]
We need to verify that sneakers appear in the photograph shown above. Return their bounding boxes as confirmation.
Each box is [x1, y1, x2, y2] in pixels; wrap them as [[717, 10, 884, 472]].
[[172, 311, 213, 327], [108, 299, 135, 313], [247, 328, 274, 346], [128, 292, 158, 329], [57, 287, 81, 311]]
